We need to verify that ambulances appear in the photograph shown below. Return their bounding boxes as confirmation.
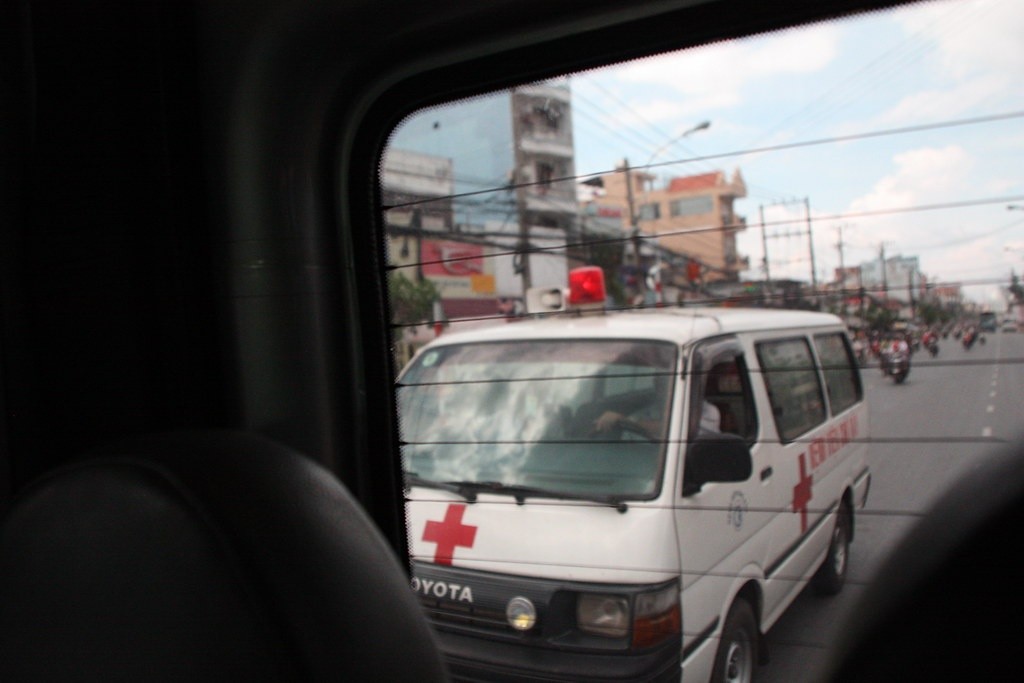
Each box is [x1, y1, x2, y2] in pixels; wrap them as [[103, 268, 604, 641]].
[[393, 264, 875, 683]]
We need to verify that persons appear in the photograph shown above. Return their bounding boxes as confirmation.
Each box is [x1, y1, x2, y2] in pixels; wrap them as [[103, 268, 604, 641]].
[[848, 329, 909, 373], [595, 366, 720, 443]]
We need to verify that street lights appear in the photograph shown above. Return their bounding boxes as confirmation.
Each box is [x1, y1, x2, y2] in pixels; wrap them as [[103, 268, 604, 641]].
[[623, 120, 713, 306]]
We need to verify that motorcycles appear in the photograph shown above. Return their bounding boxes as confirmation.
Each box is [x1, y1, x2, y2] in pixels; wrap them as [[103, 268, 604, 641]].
[[859, 312, 980, 385]]
